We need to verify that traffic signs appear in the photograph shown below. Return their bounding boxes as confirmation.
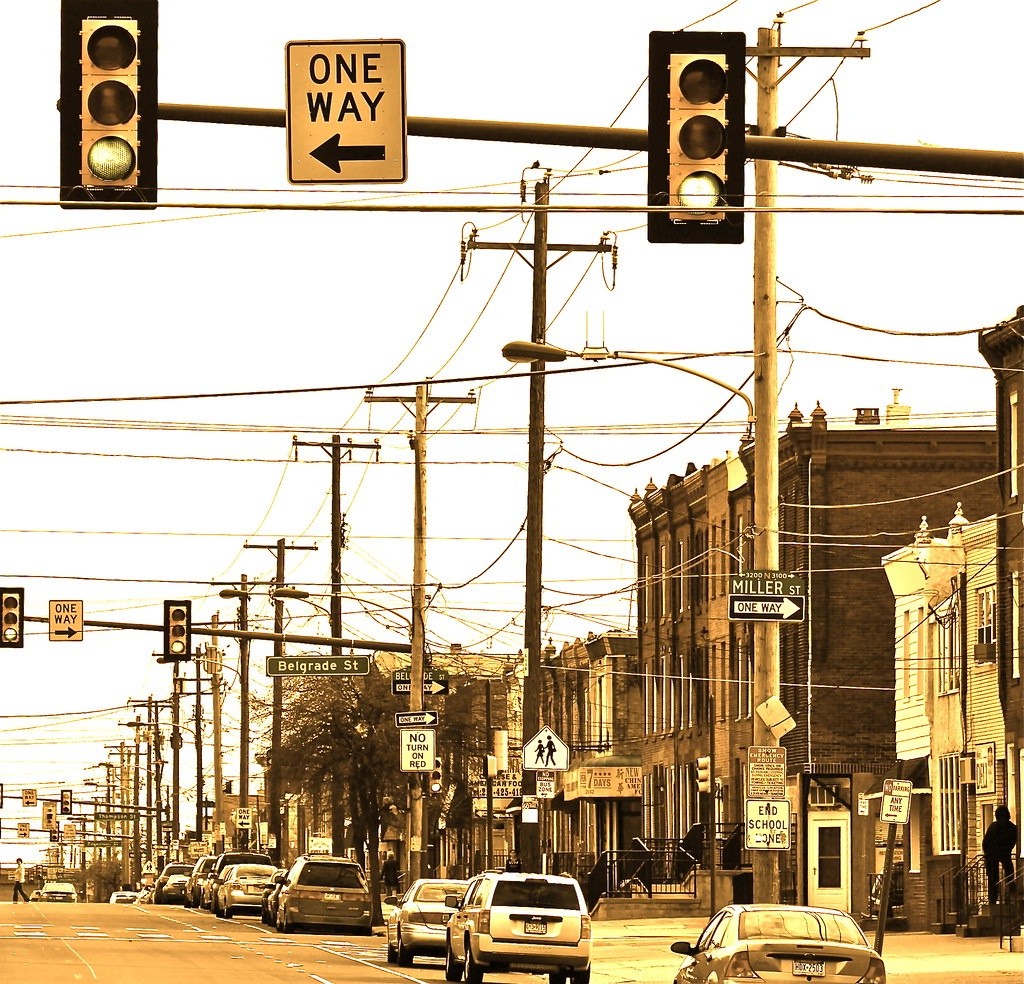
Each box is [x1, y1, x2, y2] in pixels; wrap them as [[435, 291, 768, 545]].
[[49, 600, 84, 642], [395, 711, 438, 727], [392, 669, 449, 695], [286, 38, 408, 186], [729, 571, 806, 622], [22, 789, 37, 807], [880, 778, 913, 824]]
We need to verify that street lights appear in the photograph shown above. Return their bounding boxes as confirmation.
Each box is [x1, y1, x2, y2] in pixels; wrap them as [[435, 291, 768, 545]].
[[503, 341, 781, 904], [219, 588, 424, 876], [84, 721, 204, 843]]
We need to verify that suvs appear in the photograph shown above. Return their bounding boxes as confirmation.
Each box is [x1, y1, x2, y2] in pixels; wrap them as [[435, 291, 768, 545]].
[[184, 852, 273, 909], [110, 862, 195, 904], [445, 870, 592, 984]]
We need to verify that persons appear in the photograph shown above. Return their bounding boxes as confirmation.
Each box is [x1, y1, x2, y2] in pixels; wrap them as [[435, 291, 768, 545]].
[[982, 804, 1018, 904], [380, 851, 400, 896], [506, 849, 521, 872], [13, 857, 30, 902]]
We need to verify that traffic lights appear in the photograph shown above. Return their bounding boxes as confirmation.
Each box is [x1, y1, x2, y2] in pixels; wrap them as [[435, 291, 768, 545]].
[[164, 600, 191, 661], [60, 0, 158, 210], [648, 31, 746, 243], [61, 789, 72, 815], [50, 821, 59, 842], [0, 588, 24, 648]]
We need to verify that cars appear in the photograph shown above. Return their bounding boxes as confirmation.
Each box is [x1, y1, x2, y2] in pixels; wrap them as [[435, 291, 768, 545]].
[[29, 883, 77, 903], [210, 864, 278, 918], [670, 904, 887, 984], [261, 853, 373, 936], [384, 879, 470, 967]]
